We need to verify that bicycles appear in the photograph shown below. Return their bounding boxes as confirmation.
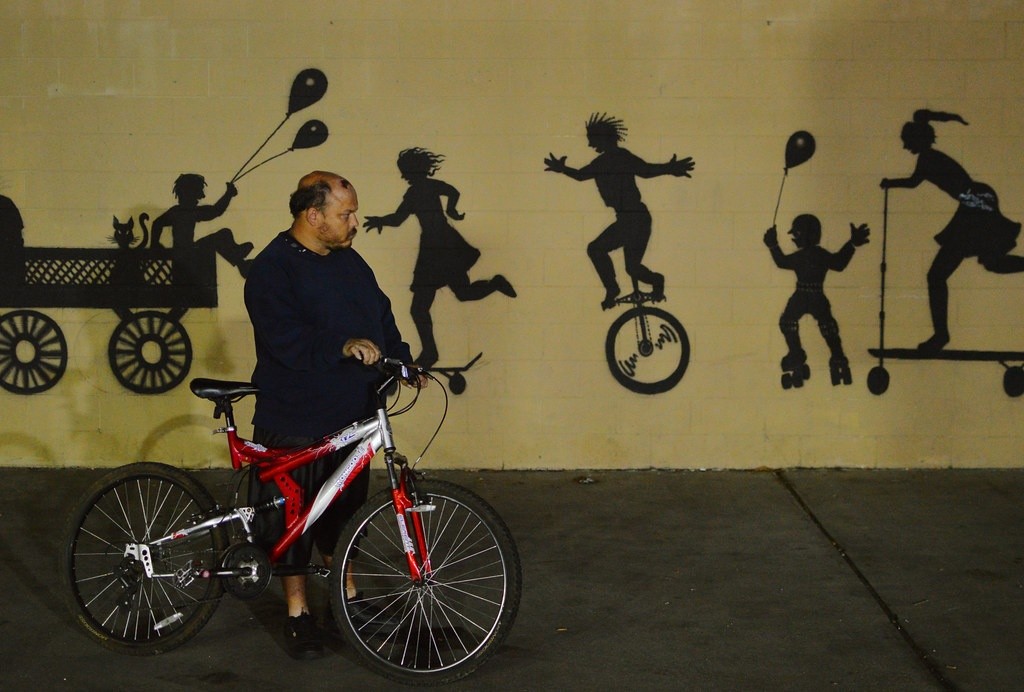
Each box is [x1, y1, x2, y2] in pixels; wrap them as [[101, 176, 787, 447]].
[[63, 341, 522, 669]]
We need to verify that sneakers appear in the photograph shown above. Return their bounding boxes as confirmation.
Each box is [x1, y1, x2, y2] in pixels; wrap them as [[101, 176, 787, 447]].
[[347, 592, 392, 624], [284, 607, 324, 659]]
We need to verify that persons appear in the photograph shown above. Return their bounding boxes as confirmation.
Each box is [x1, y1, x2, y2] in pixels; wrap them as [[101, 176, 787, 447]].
[[244, 171, 427, 658]]
